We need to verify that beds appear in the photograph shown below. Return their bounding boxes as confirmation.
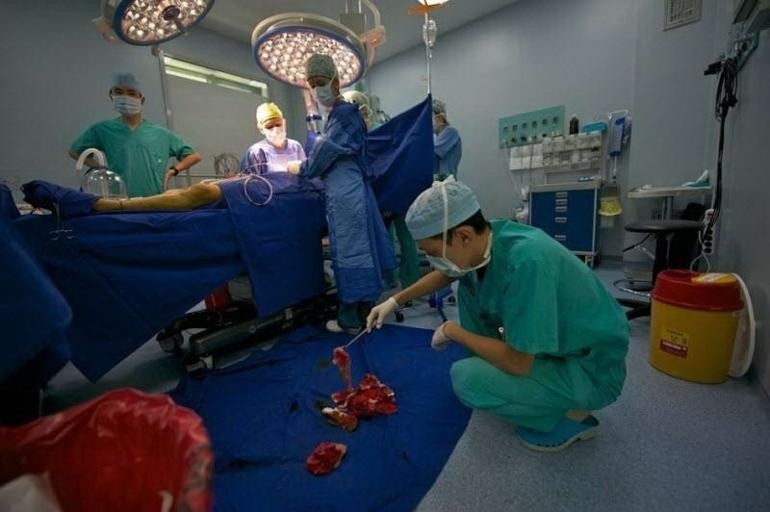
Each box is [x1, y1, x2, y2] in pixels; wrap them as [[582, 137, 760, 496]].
[[1, 92, 435, 418]]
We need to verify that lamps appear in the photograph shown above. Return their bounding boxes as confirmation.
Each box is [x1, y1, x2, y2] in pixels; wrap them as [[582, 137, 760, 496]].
[[251, 1, 381, 92], [102, 0, 216, 55]]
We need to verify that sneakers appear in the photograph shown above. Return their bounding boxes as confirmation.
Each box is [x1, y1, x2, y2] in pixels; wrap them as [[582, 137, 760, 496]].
[[513, 414, 599, 452], [325, 319, 361, 337]]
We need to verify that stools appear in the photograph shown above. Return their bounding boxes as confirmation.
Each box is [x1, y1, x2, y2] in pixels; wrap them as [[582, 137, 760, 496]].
[[612, 218, 707, 322]]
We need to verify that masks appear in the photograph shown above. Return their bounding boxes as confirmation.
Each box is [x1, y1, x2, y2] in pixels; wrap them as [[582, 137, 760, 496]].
[[430, 257, 464, 278], [112, 96, 142, 117], [263, 126, 287, 148], [310, 85, 337, 106]]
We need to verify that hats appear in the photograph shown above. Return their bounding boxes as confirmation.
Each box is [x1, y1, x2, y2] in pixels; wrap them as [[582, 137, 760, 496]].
[[255, 102, 283, 126], [107, 72, 147, 103], [303, 54, 339, 82], [431, 97, 445, 115], [404, 182, 480, 241], [341, 90, 370, 112]]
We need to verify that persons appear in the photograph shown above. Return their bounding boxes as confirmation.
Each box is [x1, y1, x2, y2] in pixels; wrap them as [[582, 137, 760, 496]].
[[365, 183, 627, 450], [23, 175, 220, 220], [287, 54, 398, 336], [68, 72, 200, 195], [345, 88, 423, 300], [432, 97, 461, 182], [242, 102, 307, 174]]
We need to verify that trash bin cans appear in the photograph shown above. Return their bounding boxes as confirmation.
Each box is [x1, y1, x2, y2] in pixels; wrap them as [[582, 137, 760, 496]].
[[650, 270, 744, 384]]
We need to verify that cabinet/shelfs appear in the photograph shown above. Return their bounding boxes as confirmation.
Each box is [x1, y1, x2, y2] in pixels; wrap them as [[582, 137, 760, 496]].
[[529, 181, 603, 267], [542, 132, 605, 174]]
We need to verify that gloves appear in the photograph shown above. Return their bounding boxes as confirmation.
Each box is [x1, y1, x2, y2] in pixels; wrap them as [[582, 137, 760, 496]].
[[366, 298, 400, 334], [431, 320, 459, 351]]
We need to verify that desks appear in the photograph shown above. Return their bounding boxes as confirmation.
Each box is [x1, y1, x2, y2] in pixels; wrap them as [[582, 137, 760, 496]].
[[628, 183, 710, 291]]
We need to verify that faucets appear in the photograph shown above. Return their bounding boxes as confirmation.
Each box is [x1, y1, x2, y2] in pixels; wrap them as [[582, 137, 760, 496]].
[[76, 147, 105, 172]]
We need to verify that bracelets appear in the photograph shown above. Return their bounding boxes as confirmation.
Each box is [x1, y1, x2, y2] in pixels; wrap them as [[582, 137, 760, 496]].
[[170, 165, 180, 179]]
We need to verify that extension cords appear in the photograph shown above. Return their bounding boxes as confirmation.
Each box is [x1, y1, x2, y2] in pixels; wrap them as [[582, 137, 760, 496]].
[[700, 208, 716, 256]]
